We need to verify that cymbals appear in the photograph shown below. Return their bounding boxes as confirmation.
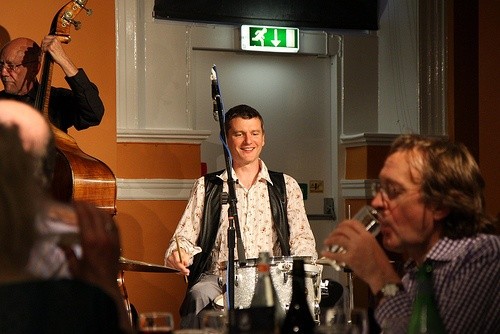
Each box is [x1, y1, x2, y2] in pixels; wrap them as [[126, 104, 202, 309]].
[[117, 255, 181, 281], [317, 252, 397, 272]]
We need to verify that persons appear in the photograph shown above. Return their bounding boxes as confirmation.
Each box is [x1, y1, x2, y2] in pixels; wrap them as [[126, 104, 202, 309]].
[[0, 35, 105, 133], [320, 132, 500, 334], [164, 104, 318, 329], [0, 100, 133, 334]]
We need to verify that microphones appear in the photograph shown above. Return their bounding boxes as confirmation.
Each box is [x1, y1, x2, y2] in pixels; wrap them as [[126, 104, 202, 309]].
[[210, 74, 220, 121]]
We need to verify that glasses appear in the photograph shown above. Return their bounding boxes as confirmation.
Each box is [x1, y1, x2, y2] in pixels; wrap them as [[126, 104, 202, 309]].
[[371, 182, 426, 203], [0, 60, 40, 72]]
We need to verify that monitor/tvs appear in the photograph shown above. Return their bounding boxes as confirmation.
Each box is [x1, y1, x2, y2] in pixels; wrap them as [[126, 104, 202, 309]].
[[152, 0, 379, 31]]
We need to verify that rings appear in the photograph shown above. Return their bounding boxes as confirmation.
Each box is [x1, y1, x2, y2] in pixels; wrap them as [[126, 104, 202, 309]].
[[106, 223, 117, 233]]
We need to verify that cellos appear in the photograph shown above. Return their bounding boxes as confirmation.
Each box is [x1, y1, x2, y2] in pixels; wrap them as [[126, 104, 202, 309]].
[[26, 2, 118, 217]]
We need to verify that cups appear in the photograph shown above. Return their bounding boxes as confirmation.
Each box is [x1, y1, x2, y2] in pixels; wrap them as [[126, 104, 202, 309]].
[[201, 311, 225, 334], [324, 205, 383, 271], [139, 312, 175, 334]]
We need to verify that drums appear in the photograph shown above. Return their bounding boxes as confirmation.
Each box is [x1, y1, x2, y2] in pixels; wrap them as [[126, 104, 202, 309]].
[[218, 255, 322, 325]]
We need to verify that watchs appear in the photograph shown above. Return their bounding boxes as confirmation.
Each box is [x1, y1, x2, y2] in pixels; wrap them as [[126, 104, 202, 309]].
[[371, 282, 406, 315]]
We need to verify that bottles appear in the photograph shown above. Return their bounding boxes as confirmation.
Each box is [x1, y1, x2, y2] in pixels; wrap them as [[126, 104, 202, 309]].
[[284, 256, 316, 334], [250, 251, 281, 334]]
